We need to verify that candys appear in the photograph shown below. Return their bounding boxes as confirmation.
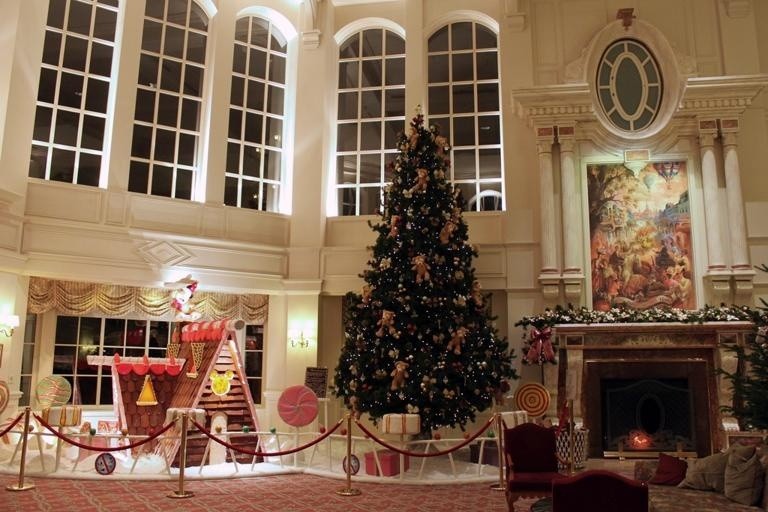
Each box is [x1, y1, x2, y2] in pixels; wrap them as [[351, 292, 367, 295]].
[[277, 385, 319, 466], [514, 381, 551, 424]]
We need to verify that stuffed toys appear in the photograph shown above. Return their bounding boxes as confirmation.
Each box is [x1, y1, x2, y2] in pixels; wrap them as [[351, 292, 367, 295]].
[[348, 106, 508, 421], [170, 281, 202, 321]]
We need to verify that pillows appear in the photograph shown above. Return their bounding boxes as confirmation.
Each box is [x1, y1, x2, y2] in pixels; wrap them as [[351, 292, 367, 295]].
[[649, 442, 764, 506]]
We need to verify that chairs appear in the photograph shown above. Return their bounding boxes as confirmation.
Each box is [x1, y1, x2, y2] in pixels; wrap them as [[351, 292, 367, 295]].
[[504, 422, 648, 512]]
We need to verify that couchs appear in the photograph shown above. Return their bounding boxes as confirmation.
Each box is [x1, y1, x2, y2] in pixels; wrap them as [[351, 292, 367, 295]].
[[634, 454, 768, 512]]
